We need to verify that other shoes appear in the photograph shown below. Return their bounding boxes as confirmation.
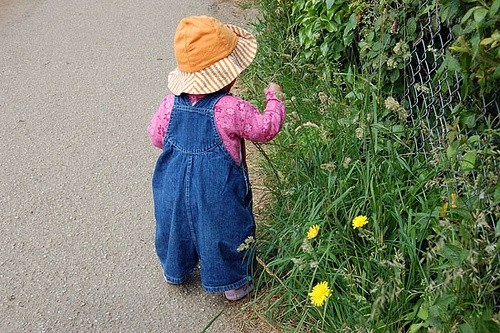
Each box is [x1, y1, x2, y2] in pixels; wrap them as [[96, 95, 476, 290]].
[[225, 283, 253, 300]]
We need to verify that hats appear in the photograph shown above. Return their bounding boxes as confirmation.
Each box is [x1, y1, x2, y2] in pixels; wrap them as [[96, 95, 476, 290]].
[[168, 15, 258, 96]]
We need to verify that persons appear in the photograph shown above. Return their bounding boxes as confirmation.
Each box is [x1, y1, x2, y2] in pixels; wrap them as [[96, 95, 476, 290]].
[[149, 15, 285, 301]]
